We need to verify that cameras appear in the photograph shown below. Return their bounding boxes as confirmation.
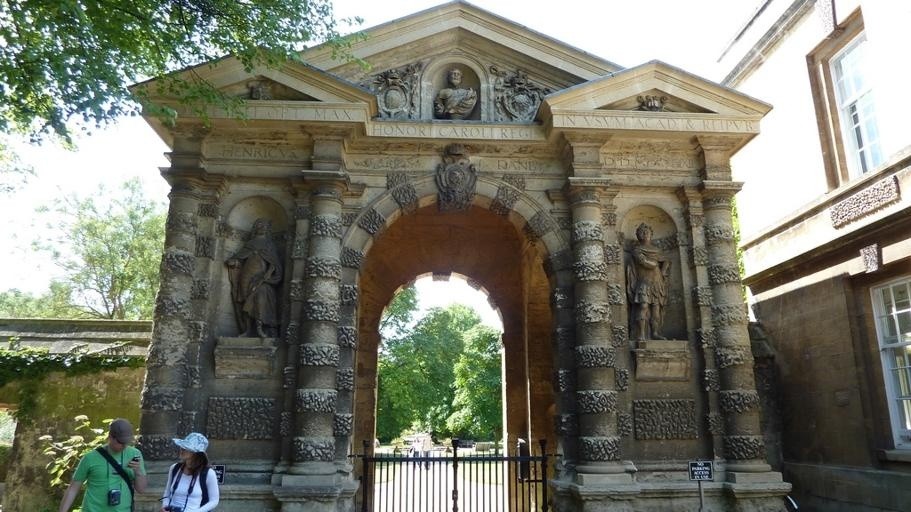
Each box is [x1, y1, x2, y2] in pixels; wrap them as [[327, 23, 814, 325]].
[[108, 489, 121, 505], [166, 505, 183, 512]]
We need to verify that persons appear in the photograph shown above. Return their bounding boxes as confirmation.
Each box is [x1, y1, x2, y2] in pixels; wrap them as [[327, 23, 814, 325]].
[[410, 432, 433, 472], [60, 420, 148, 511], [159, 432, 220, 512], [626, 223, 670, 341], [434, 67, 480, 120], [225, 219, 283, 337]]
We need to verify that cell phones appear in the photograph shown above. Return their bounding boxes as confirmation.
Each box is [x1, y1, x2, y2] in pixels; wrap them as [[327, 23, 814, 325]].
[[132, 456, 140, 462]]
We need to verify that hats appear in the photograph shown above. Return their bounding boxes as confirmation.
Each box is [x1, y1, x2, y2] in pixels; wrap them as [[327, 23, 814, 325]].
[[171, 432, 209, 453], [109, 418, 133, 443]]
